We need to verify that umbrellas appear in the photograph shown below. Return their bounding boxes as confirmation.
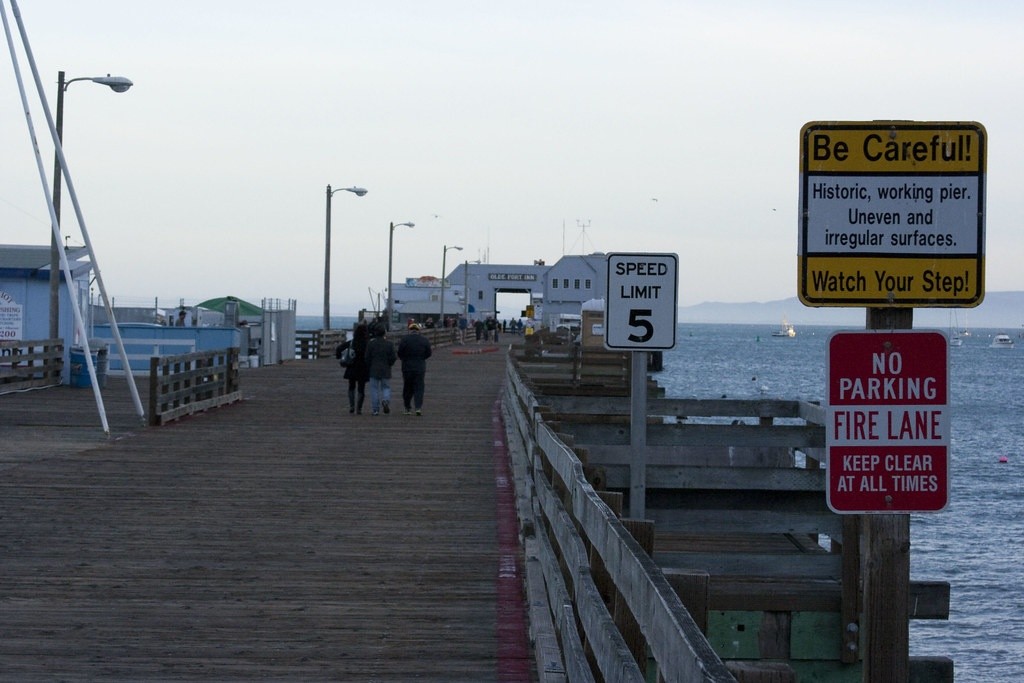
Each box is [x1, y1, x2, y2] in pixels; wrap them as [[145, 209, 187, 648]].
[[196, 294, 264, 316]]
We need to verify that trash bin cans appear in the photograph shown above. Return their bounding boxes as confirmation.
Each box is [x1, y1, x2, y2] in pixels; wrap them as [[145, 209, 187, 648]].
[[89, 338, 110, 387], [556, 326, 569, 334], [69, 346, 99, 388]]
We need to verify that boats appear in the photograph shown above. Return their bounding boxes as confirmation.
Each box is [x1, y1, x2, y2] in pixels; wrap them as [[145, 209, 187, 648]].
[[771, 319, 796, 338], [988, 334, 1014, 350]]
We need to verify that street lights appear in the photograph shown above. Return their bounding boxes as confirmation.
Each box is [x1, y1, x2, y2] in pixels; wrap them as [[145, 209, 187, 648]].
[[439, 245, 464, 328], [322, 183, 369, 329], [48, 70, 134, 340], [463, 258, 482, 330], [386, 221, 418, 331]]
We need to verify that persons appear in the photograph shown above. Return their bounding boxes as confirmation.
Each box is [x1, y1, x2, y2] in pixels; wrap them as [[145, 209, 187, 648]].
[[176, 311, 185, 327], [367, 325, 397, 415], [475, 317, 483, 340], [398, 323, 432, 414], [509, 317, 517, 335], [484, 315, 499, 344], [503, 318, 506, 334], [335, 325, 371, 415], [517, 319, 523, 336], [408, 317, 458, 330], [459, 314, 467, 345]]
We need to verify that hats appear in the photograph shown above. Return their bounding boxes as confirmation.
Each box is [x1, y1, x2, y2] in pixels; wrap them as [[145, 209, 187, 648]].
[[409, 323, 420, 329]]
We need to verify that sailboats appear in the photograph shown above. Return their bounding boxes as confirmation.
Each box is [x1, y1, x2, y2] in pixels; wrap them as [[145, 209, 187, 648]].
[[947, 308, 964, 347], [959, 309, 971, 337]]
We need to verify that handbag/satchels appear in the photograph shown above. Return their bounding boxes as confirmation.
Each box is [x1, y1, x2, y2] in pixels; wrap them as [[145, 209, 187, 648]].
[[340, 347, 355, 367]]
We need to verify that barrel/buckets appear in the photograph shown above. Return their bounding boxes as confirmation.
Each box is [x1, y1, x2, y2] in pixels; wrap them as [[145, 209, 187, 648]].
[[69, 346, 98, 387], [239, 355, 249, 369], [250, 355, 260, 368], [97, 343, 112, 386]]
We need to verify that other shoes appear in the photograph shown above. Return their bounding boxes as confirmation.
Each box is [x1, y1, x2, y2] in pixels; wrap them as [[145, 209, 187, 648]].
[[372, 411, 379, 415], [403, 409, 411, 414], [357, 408, 362, 414], [416, 408, 422, 416], [383, 400, 389, 414], [349, 408, 355, 413]]
